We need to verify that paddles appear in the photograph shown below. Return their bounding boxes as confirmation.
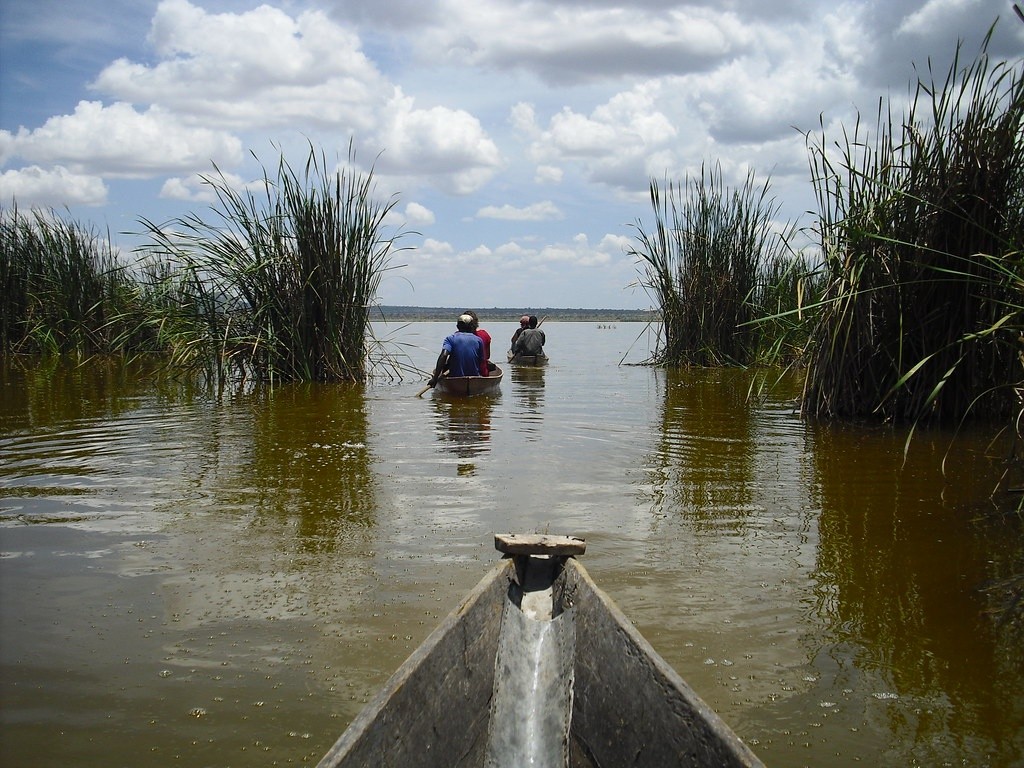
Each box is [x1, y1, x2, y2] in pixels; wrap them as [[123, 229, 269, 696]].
[[413, 370, 451, 397], [508, 316, 547, 364]]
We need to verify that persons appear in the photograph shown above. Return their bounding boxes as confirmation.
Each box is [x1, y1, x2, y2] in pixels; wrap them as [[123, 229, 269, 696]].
[[510, 316, 545, 356], [427, 310, 491, 388]]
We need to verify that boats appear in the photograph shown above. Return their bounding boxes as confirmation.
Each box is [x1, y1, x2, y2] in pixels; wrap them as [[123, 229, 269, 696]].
[[506, 348, 550, 365], [431, 359, 505, 397]]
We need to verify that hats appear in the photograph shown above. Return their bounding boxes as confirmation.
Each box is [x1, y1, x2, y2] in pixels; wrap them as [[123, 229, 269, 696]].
[[458, 315, 473, 325], [520, 316, 529, 324]]
